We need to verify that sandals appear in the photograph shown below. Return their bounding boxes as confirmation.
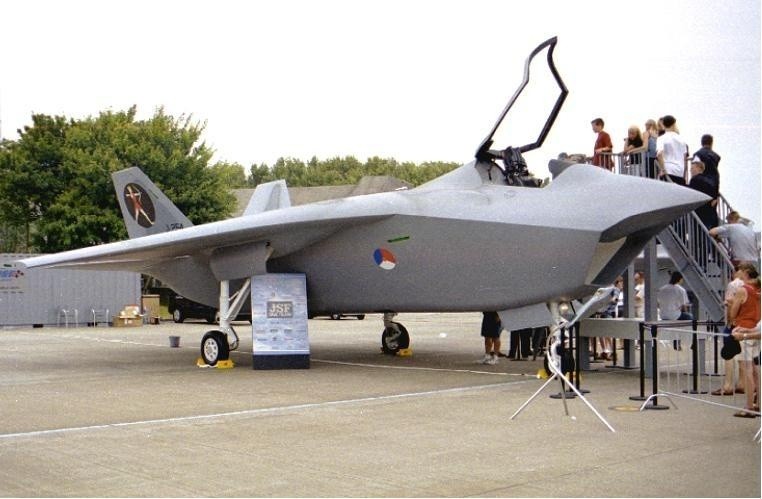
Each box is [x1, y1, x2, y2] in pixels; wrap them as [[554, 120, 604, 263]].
[[733, 405, 760, 418]]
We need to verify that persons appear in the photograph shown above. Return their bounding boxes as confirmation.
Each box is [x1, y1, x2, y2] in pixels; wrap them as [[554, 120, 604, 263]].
[[481, 311, 547, 365], [558, 152, 586, 164], [711, 264, 761, 417], [384, 310, 401, 346], [657, 271, 693, 321], [709, 211, 761, 268], [589, 272, 645, 361], [618, 115, 721, 272], [589, 118, 613, 172], [121, 185, 154, 225]]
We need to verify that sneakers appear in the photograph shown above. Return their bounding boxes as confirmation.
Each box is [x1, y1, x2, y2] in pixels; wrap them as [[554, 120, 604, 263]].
[[602, 351, 617, 360], [478, 356, 499, 365], [711, 385, 745, 396]]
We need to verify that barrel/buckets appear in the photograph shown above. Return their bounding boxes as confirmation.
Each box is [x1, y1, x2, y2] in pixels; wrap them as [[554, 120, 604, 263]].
[[167, 335, 181, 350]]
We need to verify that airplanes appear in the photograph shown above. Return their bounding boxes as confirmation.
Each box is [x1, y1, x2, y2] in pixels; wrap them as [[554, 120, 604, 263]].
[[14, 35, 713, 380]]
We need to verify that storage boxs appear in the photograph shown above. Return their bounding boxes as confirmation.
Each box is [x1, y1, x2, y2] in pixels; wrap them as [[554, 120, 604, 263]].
[[112, 312, 143, 327]]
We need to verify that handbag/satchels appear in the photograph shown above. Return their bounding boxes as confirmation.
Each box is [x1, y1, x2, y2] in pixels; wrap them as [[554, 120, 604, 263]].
[[721, 335, 741, 360]]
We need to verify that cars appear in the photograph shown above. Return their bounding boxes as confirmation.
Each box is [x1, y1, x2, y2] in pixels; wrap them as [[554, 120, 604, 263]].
[[167, 287, 252, 324]]
